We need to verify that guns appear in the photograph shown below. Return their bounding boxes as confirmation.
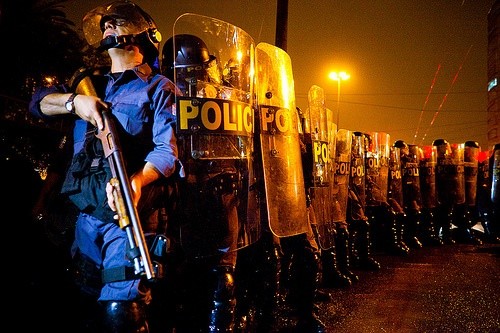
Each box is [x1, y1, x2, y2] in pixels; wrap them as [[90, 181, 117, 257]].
[[66, 66, 156, 282]]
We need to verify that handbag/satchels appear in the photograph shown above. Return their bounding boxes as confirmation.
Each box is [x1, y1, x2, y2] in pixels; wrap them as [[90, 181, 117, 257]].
[[59, 151, 134, 230]]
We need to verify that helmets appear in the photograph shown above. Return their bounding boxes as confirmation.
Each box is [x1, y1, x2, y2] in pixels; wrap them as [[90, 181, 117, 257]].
[[465, 140, 481, 148], [161, 33, 218, 78], [393, 140, 407, 148], [432, 138, 448, 146], [364, 132, 372, 139], [353, 131, 363, 136], [96, 1, 162, 57]]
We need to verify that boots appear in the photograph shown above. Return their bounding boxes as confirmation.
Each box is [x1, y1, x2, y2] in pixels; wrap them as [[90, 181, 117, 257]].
[[79, 204, 499, 332]]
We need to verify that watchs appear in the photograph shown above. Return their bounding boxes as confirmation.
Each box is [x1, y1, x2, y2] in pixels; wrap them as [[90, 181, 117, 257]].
[[65, 92, 79, 114]]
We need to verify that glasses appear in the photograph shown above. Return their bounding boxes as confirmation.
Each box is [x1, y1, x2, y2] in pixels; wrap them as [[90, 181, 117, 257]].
[[107, 16, 127, 25]]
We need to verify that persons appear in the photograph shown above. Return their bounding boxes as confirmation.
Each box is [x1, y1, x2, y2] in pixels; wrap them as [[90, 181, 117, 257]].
[[28, 0, 500, 333]]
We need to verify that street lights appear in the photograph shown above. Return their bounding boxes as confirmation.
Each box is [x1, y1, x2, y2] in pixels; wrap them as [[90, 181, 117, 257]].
[[328, 71, 350, 131]]
